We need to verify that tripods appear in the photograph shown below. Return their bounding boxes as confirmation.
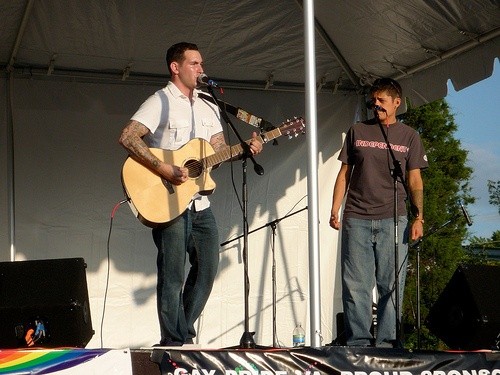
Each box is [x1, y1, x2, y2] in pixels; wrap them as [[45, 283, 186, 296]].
[[207, 85, 281, 349]]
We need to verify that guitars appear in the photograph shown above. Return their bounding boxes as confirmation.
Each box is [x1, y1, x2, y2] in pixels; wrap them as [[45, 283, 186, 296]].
[[120, 114, 306, 230]]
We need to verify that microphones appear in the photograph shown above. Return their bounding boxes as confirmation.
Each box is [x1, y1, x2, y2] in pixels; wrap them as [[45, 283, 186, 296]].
[[366, 101, 387, 113], [198, 74, 218, 88], [460, 204, 472, 226]]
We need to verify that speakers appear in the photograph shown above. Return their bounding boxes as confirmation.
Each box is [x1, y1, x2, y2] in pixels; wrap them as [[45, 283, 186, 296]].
[[0, 257, 95, 348], [424, 265, 500, 352]]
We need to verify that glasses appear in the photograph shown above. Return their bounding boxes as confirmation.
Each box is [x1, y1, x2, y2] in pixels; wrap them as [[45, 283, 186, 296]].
[[373, 78, 401, 98]]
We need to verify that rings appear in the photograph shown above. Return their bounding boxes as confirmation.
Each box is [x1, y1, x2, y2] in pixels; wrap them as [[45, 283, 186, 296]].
[[255, 150, 259, 154]]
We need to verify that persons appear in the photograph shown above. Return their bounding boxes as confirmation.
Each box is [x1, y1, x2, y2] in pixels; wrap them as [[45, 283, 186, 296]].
[[329, 77, 430, 347], [119, 42, 263, 347]]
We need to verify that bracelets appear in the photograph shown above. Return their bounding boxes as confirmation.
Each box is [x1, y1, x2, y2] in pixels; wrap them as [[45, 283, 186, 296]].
[[416, 218, 424, 224]]
